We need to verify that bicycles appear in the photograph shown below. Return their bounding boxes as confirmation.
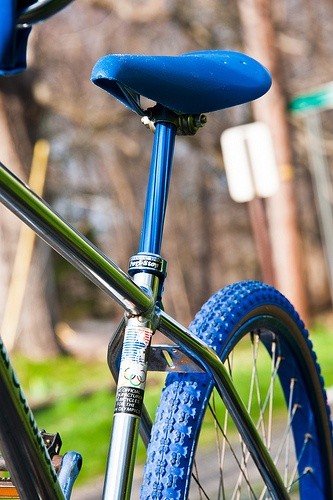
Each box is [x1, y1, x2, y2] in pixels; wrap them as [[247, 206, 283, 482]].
[[0, 0, 331, 499]]
